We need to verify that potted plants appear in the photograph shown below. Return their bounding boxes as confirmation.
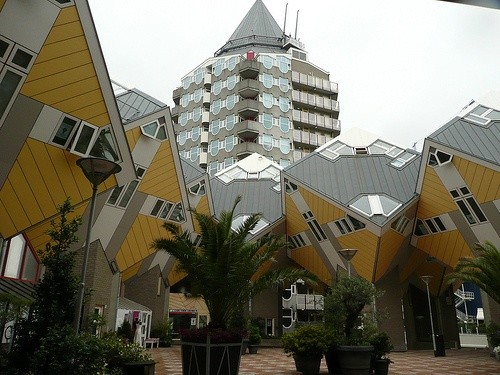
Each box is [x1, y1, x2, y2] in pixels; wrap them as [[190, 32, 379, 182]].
[[280, 273, 395, 375], [179, 334, 244, 375], [240, 320, 262, 355]]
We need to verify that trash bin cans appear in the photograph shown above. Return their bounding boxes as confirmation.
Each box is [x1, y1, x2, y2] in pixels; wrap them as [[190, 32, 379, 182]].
[[435, 335, 445, 357]]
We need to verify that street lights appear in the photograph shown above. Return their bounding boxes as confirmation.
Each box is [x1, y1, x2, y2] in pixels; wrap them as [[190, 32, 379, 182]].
[[419, 276, 438, 353], [75, 156, 122, 340], [337, 249, 359, 279]]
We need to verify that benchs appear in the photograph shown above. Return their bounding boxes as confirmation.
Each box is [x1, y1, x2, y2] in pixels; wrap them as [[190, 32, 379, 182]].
[[145, 337, 159, 350]]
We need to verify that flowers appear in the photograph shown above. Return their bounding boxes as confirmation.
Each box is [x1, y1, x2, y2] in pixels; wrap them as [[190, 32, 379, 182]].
[[148, 192, 308, 345]]
